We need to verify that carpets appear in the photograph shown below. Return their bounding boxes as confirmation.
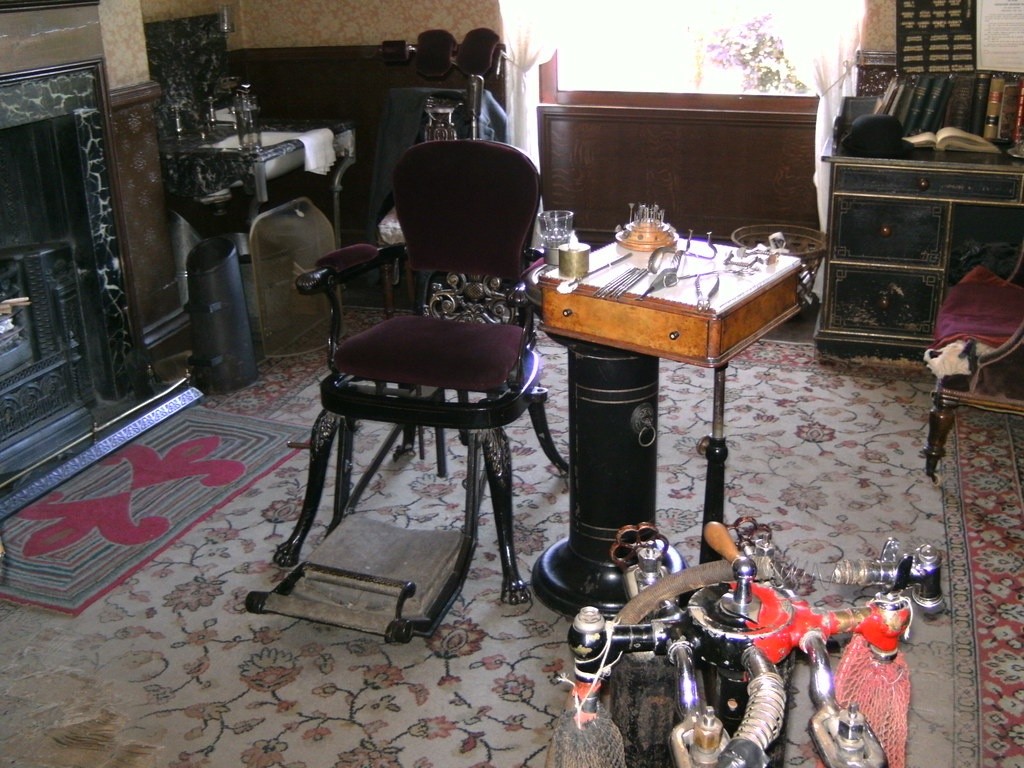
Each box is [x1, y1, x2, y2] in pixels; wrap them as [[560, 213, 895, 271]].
[[0, 405, 311, 615]]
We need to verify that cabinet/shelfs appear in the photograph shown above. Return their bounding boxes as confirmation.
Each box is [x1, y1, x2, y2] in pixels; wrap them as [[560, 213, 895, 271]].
[[537, 91, 822, 326], [813, 136, 1024, 369]]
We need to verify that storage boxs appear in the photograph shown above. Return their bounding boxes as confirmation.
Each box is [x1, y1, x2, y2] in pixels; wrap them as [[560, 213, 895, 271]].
[[533, 239, 806, 369]]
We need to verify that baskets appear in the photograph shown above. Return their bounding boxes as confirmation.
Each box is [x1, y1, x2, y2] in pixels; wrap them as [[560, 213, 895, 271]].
[[731, 224, 827, 313]]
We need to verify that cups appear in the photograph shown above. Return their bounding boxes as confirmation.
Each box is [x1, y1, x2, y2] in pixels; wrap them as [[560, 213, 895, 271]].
[[537, 210, 574, 248]]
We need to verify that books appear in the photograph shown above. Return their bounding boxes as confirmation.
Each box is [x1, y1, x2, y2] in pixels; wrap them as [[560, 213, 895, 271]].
[[873, 70, 1024, 153]]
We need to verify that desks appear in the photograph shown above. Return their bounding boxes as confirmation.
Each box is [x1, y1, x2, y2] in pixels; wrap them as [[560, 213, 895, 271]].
[[159, 118, 361, 311]]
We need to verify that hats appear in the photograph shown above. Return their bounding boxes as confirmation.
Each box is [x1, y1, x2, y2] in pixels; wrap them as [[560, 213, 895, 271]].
[[841, 114, 915, 159]]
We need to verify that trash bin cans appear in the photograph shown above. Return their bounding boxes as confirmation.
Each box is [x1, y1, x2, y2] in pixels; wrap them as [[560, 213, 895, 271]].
[[183, 236, 260, 394], [731, 221, 827, 313]]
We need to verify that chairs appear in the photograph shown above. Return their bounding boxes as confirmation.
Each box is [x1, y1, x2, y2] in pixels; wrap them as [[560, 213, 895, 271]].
[[921, 244, 1024, 477], [238, 137, 570, 646]]
